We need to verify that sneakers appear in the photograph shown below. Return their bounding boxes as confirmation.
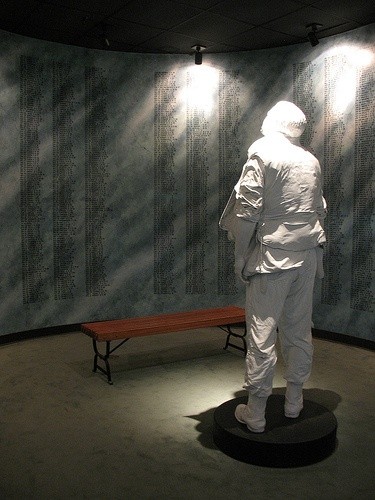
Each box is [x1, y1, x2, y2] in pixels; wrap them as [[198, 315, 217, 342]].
[[235, 402, 267, 432], [284, 393, 305, 418]]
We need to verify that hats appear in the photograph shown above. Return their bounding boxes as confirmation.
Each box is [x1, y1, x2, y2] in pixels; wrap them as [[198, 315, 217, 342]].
[[263, 100, 307, 138]]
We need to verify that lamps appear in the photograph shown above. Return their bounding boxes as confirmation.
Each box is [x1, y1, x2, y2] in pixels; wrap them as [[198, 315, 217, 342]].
[[190, 44, 206, 65], [306, 23, 323, 47]]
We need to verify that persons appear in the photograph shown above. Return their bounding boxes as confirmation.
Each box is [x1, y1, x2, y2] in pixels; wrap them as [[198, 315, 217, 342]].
[[219, 100, 329, 436]]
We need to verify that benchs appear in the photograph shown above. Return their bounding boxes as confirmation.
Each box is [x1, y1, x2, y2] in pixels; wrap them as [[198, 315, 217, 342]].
[[80, 306, 246, 385]]
